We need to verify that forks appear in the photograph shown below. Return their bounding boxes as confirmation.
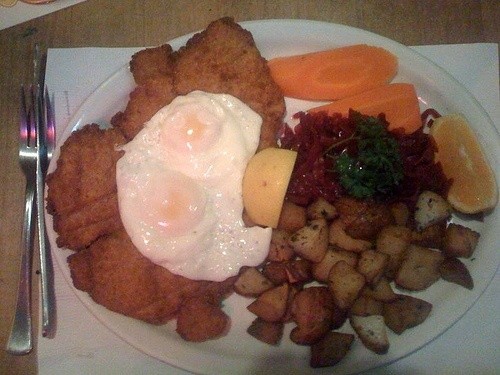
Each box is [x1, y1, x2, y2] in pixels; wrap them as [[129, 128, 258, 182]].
[[6, 83, 55, 356]]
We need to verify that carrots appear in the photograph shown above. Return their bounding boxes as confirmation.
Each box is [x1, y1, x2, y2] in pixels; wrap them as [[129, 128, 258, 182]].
[[267, 45, 398, 99], [313, 83, 422, 129]]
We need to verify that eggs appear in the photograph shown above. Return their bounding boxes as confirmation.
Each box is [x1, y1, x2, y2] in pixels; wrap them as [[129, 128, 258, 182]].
[[116, 90, 272, 283]]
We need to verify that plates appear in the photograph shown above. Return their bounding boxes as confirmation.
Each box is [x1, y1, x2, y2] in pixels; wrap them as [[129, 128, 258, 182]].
[[40, 18, 500, 373]]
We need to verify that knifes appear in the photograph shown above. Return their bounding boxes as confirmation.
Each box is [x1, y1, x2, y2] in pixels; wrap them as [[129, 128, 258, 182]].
[[32, 85, 56, 342]]
